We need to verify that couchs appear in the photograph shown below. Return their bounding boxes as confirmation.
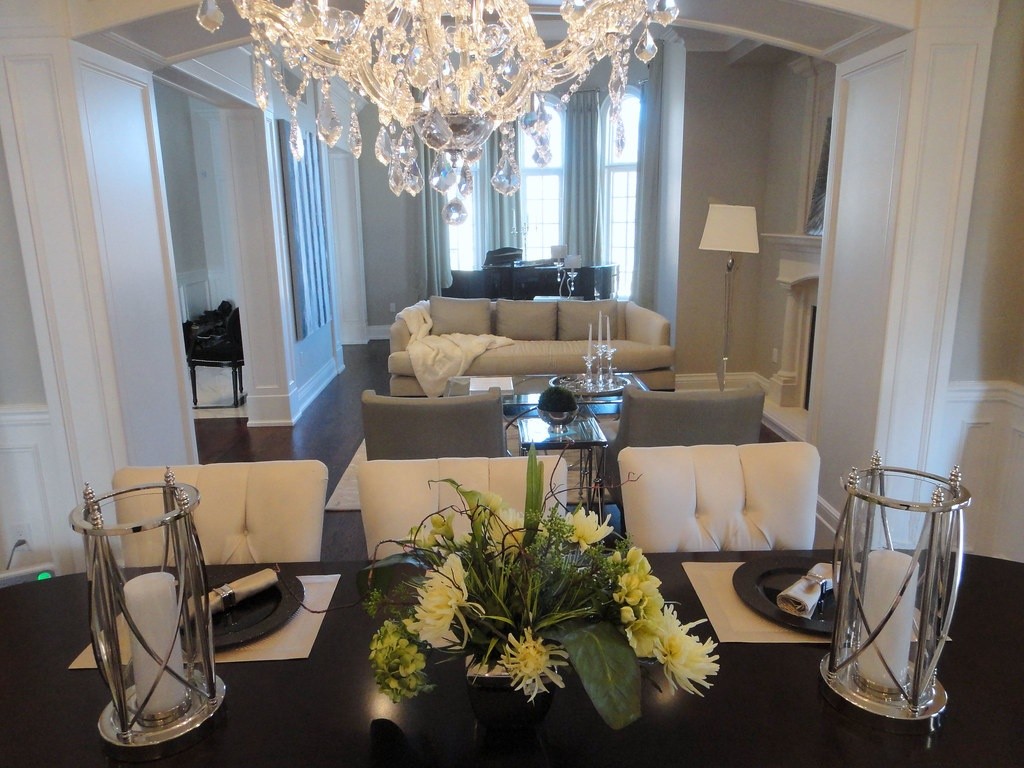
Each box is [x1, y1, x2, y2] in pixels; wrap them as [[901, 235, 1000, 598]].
[[387, 295, 677, 392], [595, 390, 766, 511], [362, 386, 514, 458]]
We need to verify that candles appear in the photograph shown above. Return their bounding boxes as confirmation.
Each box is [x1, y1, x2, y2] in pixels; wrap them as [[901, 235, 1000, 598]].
[[854, 550, 921, 688], [122, 571, 186, 712]]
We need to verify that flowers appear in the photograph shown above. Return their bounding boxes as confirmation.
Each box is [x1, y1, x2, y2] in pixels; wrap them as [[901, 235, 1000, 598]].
[[357, 475, 720, 730]]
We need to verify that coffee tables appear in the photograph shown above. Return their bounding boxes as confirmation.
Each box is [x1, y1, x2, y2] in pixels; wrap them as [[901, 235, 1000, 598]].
[[441, 373, 652, 433]]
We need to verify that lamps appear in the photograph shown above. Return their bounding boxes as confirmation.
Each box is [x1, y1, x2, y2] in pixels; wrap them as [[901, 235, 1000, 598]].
[[549, 246, 582, 300], [698, 204, 760, 392], [197, 0, 679, 220]]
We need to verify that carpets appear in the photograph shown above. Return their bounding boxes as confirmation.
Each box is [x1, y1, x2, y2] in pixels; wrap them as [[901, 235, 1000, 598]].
[[325, 438, 370, 511], [513, 394, 608, 515]]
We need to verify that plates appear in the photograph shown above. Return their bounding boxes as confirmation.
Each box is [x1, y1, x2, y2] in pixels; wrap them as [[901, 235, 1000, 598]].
[[207, 569, 305, 648], [732, 554, 838, 633]]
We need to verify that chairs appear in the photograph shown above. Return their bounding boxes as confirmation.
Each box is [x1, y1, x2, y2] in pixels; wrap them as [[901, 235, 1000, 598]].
[[112, 460, 328, 566], [186, 307, 248, 409], [354, 455, 567, 559], [616, 441, 822, 552]]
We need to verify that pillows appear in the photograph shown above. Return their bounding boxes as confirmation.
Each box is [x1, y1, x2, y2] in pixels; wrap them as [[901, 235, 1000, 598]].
[[497, 299, 556, 341], [430, 295, 491, 336], [556, 300, 618, 341]]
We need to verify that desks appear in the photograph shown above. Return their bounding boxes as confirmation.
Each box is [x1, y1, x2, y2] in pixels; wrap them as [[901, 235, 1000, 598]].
[[439, 266, 614, 300], [0, 534, 1024, 768], [533, 295, 583, 301]]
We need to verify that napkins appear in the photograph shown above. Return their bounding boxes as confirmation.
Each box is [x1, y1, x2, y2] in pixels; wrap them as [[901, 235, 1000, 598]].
[[779, 562, 841, 620], [177, 566, 277, 627]]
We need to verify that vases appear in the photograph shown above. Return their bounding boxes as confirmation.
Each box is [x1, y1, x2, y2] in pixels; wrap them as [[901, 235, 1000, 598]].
[[463, 652, 558, 735]]
